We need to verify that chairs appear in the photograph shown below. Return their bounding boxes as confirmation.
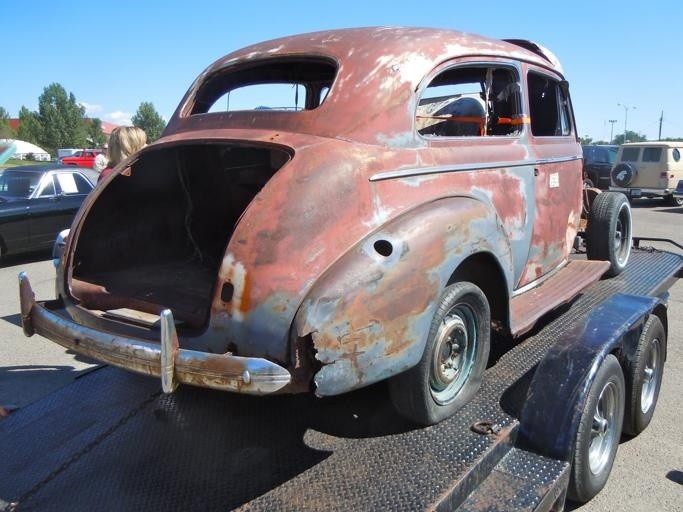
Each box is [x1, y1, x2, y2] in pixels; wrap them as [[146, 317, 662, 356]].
[[7, 179, 30, 196]]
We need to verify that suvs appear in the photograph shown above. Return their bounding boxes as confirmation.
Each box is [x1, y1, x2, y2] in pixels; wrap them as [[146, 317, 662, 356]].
[[580, 142, 682, 207]]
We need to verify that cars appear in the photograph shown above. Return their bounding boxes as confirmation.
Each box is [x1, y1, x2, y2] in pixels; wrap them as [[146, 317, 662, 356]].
[[53, 149, 107, 169], [0, 164, 96, 260]]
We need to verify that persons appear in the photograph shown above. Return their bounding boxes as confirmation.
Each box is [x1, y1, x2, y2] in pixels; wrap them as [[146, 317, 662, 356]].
[[93, 142, 110, 173], [96, 126, 149, 184]]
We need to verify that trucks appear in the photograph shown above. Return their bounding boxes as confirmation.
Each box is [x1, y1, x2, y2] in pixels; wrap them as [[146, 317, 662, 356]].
[[1, 237, 683, 511]]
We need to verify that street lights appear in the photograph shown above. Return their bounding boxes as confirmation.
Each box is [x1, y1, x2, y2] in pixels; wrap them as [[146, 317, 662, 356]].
[[608, 119, 617, 142], [618, 103, 636, 143]]
